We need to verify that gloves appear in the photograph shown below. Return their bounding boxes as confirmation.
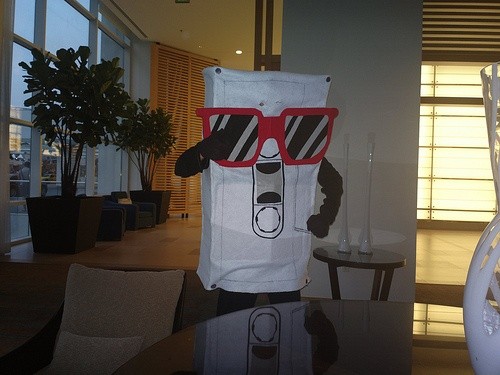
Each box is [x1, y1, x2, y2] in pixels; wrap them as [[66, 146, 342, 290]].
[[307, 214, 329, 238], [198, 128, 225, 157]]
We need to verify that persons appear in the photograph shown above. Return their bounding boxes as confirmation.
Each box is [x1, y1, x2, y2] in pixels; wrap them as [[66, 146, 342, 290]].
[[174, 67, 343, 317], [17, 162, 31, 197]]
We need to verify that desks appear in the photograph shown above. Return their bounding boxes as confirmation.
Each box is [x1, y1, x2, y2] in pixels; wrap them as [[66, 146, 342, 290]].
[[111, 300, 500, 375], [312, 246, 407, 300]]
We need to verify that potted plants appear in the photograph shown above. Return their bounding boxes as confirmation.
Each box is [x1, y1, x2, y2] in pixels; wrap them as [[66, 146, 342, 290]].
[[19, 45, 139, 256], [113, 97, 179, 225]]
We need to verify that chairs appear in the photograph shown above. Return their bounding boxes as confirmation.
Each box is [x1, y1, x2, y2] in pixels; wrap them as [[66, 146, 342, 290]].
[[463, 300, 500, 375], [0, 263, 187, 375], [78, 191, 157, 242]]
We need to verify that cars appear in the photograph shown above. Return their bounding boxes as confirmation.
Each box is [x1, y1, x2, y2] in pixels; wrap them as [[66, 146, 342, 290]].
[[9, 149, 60, 182], [9, 173, 48, 201]]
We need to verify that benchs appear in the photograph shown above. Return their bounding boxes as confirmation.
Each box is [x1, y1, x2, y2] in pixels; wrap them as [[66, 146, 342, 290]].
[[463, 210, 500, 300]]
